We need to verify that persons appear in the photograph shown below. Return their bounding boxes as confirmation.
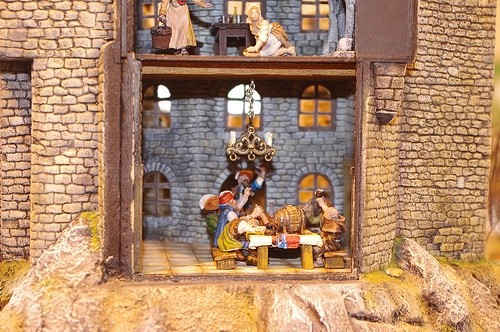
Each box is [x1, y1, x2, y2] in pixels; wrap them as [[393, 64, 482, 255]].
[[158, 0, 214, 55], [302, 188, 347, 269], [243, 3, 296, 58], [213, 187, 258, 266], [198, 194, 221, 236], [235, 166, 266, 214]]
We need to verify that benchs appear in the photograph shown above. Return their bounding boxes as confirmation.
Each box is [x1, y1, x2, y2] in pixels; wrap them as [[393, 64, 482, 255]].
[[324, 248, 347, 268], [211, 247, 238, 269]]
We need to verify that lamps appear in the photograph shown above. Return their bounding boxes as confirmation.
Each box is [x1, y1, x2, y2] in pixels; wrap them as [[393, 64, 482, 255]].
[[225, 79, 276, 162]]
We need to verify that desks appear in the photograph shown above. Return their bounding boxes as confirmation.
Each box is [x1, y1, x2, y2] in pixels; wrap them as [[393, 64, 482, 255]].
[[210, 22, 254, 57], [247, 229, 323, 269]]
